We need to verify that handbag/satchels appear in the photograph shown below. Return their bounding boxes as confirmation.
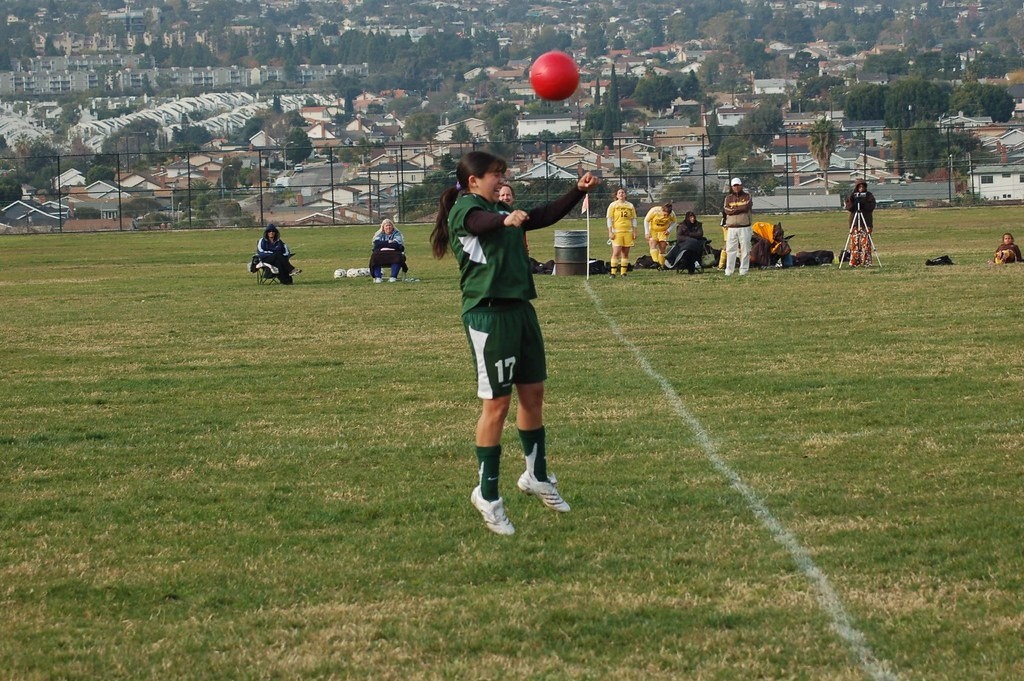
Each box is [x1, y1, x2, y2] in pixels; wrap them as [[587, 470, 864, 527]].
[[701, 243, 715, 267]]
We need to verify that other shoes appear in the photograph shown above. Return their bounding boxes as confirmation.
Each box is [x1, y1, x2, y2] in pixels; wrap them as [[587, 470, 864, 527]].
[[621, 273, 627, 278], [372, 277, 384, 283], [289, 268, 302, 276], [609, 274, 615, 279], [658, 266, 664, 271], [388, 277, 397, 283], [650, 262, 660, 268]]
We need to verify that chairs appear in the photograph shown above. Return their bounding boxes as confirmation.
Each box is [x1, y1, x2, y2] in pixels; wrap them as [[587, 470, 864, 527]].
[[752, 222, 791, 266], [252, 241, 279, 284], [380, 245, 407, 281], [666, 235, 713, 274]]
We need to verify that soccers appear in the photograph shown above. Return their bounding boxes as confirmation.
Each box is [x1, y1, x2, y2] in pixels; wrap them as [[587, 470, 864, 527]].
[[347, 268, 384, 277], [528, 50, 580, 100], [333, 268, 346, 278]]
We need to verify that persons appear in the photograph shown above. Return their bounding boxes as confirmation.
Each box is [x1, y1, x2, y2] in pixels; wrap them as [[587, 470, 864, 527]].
[[717, 175, 753, 278], [643, 204, 677, 272], [248, 224, 303, 286], [369, 218, 408, 283], [674, 211, 708, 275], [987, 232, 1024, 265], [606, 187, 639, 280], [497, 183, 529, 256], [846, 178, 877, 269], [751, 218, 792, 270], [428, 152, 603, 536]]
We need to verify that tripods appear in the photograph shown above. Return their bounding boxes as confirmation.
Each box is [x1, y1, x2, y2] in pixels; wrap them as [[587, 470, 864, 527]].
[[839, 198, 883, 270]]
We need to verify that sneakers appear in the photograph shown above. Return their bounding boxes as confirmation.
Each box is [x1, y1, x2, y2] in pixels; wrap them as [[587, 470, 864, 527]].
[[471, 485, 515, 536], [517, 470, 570, 513]]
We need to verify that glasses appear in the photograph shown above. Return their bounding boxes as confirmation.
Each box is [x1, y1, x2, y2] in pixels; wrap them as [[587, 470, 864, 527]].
[[268, 230, 275, 232]]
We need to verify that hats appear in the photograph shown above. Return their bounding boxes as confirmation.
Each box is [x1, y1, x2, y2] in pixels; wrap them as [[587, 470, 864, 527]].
[[731, 177, 742, 187]]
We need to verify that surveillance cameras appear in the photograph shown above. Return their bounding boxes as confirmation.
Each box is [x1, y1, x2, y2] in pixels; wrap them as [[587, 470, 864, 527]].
[[855, 192, 866, 198]]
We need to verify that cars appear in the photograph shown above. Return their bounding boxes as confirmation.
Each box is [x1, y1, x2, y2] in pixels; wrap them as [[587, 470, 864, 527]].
[[697, 148, 711, 157], [686, 156, 695, 165], [315, 153, 329, 159], [666, 171, 682, 187], [293, 163, 304, 173], [678, 163, 693, 175], [717, 169, 730, 179], [325, 156, 338, 163]]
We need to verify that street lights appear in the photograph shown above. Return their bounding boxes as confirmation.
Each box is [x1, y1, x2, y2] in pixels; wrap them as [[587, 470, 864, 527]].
[[171, 177, 184, 218], [284, 141, 294, 170], [177, 202, 182, 223], [440, 111, 448, 126], [221, 164, 233, 199]]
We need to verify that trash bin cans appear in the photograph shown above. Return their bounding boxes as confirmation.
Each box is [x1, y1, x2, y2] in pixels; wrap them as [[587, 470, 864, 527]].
[[553, 229, 588, 278]]
